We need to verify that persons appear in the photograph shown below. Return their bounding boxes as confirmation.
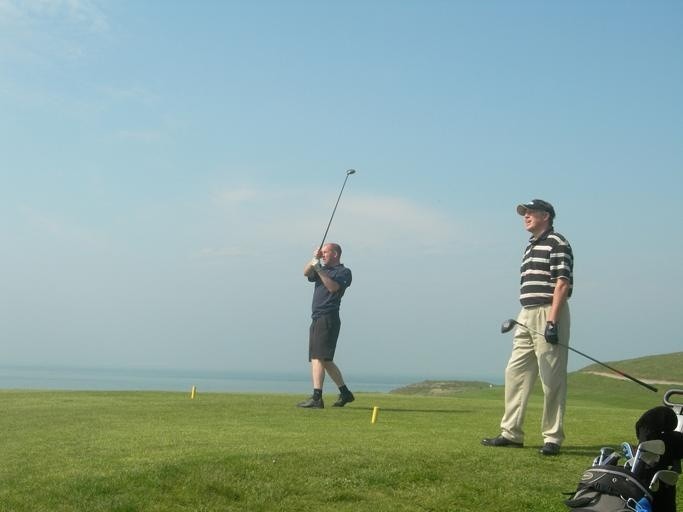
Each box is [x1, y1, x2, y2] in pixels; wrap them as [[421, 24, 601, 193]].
[[295, 242, 355, 408], [482, 197, 575, 456]]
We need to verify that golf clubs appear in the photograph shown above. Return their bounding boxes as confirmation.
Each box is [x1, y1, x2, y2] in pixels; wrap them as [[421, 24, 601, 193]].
[[317, 170, 356, 258], [501, 319, 658, 392], [593, 439, 679, 499]]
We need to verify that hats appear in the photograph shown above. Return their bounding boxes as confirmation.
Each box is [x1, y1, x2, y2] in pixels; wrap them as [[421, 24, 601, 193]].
[[517, 200, 555, 217]]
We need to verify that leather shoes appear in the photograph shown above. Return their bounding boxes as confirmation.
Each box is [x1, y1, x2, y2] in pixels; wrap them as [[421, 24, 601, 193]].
[[297, 398, 324, 408], [481, 436, 523, 447], [333, 391, 354, 407], [540, 442, 560, 455]]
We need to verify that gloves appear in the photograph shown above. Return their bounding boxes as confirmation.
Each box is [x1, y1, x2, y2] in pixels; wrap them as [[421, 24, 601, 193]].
[[544, 321, 558, 344], [311, 249, 322, 272]]
